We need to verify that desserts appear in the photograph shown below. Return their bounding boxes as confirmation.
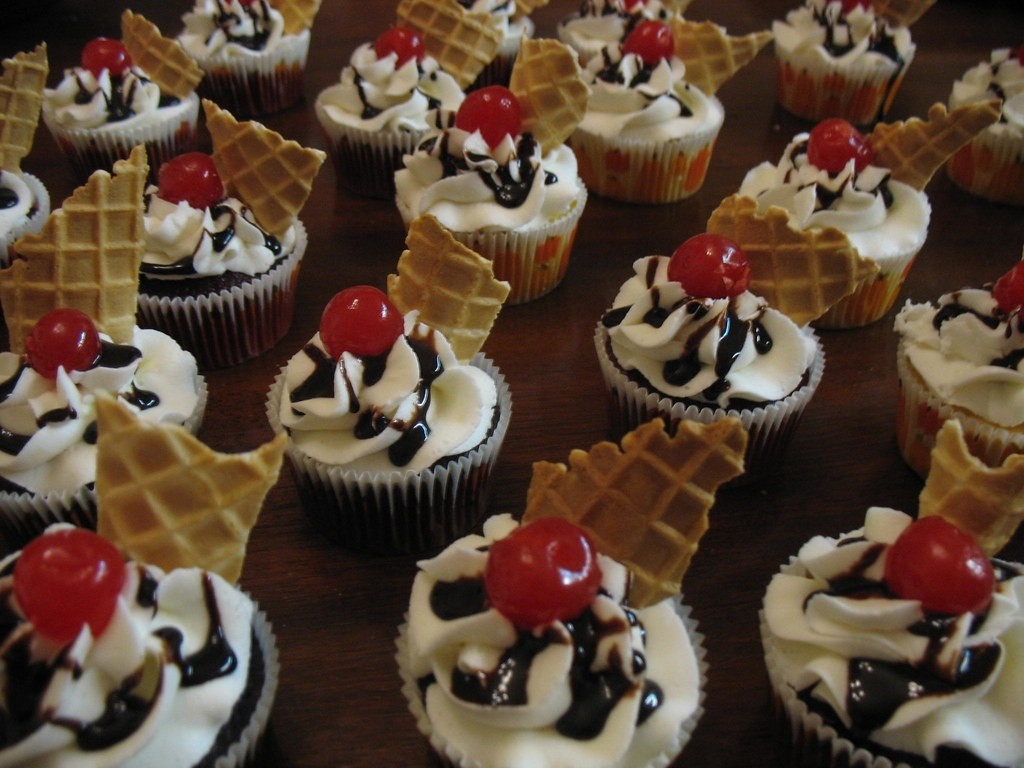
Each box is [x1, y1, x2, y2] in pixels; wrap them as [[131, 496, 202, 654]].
[[0, 0, 1024, 768]]
